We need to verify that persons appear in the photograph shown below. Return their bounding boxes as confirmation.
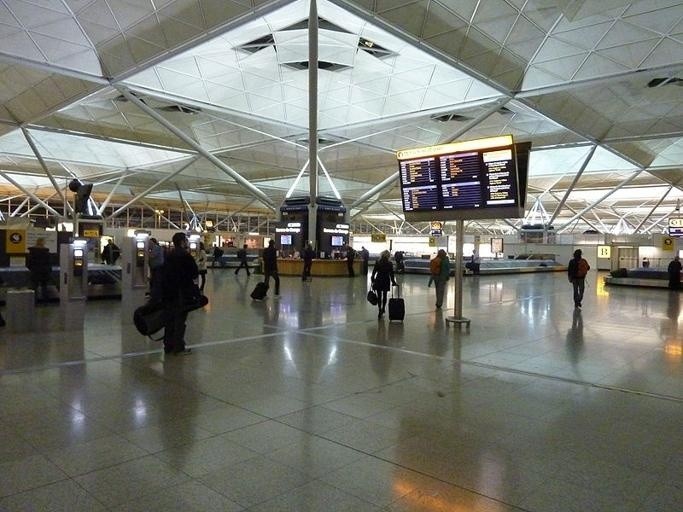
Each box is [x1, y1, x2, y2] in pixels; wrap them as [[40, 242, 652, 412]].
[[302, 244, 313, 275], [235, 244, 250, 276], [568, 249, 590, 309], [428, 249, 450, 308], [146, 232, 209, 355], [371, 250, 396, 320], [211, 244, 226, 271], [262, 239, 280, 298], [101, 239, 120, 265], [359, 247, 369, 275], [346, 247, 355, 277], [395, 251, 405, 273], [472, 249, 481, 275], [26, 238, 51, 307], [668, 256, 681, 292], [331, 247, 340, 259], [290, 247, 301, 260]]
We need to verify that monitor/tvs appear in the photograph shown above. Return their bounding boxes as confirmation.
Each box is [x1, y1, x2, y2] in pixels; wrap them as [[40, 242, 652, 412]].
[[332, 236, 343, 246], [281, 235, 292, 245]]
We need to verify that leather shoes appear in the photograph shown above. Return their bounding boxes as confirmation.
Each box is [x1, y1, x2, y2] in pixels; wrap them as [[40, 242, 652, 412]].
[[175, 349, 192, 356]]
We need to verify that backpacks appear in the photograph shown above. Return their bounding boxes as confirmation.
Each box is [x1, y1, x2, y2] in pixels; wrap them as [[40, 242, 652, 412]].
[[237, 251, 243, 258]]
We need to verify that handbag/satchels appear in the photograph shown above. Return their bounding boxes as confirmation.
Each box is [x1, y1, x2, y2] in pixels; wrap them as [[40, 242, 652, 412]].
[[135, 298, 174, 342], [367, 281, 378, 305], [188, 284, 209, 311], [431, 256, 442, 275], [576, 259, 588, 278]]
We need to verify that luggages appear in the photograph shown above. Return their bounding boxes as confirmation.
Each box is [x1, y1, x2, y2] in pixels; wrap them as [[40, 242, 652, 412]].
[[250, 282, 269, 300], [388, 285, 405, 320]]
